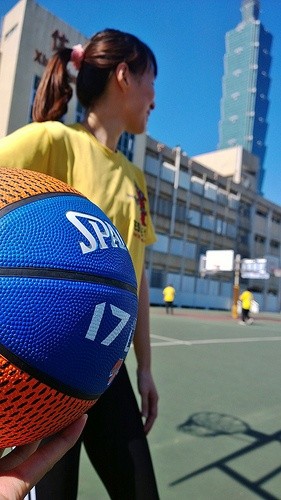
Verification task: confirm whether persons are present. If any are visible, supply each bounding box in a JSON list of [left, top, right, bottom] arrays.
[[163, 282, 175, 316], [0, 26, 161, 500], [235, 287, 254, 326]]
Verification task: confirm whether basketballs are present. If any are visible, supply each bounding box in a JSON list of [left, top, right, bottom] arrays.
[[1, 166, 141, 449]]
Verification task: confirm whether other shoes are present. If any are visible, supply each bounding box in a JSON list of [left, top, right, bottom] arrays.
[[241, 321, 246, 326], [248, 320, 252, 324]]
